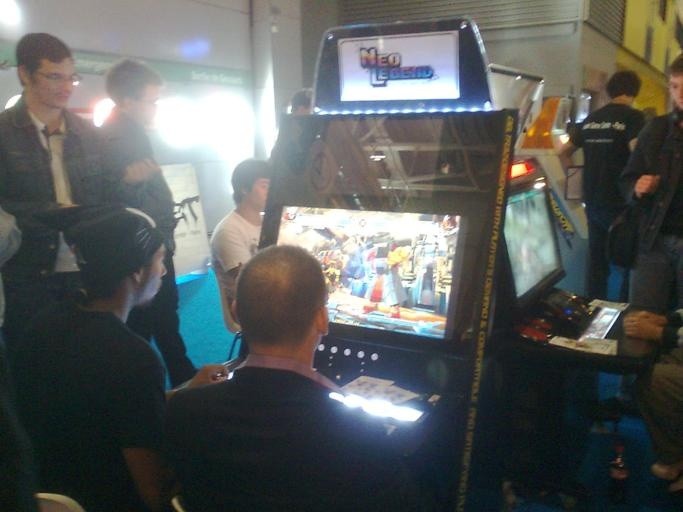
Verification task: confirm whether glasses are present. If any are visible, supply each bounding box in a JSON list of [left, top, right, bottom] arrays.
[[35, 71, 82, 86]]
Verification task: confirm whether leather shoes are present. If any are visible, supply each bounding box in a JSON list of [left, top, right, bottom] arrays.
[[650, 459, 683, 482]]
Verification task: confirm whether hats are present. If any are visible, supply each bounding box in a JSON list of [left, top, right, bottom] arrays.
[[72, 208, 184, 285]]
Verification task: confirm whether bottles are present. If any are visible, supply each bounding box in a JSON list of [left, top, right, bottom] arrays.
[[607, 448, 632, 497]]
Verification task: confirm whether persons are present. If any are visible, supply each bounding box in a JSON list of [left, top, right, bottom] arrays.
[[102, 58, 198, 390], [0, 32, 129, 344], [16, 208, 228, 512], [292, 90, 313, 114], [209, 158, 272, 334], [618, 53, 683, 310], [162, 245, 400, 512], [556, 70, 645, 303], [623, 307, 683, 492]]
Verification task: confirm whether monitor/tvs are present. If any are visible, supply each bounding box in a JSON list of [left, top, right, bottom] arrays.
[[504, 190, 560, 299], [276, 206, 460, 339]]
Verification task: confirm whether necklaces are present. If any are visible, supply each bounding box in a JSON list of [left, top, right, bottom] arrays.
[[613, 98, 633, 103]]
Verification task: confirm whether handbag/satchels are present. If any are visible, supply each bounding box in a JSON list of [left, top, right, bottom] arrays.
[[606, 204, 637, 250]]
